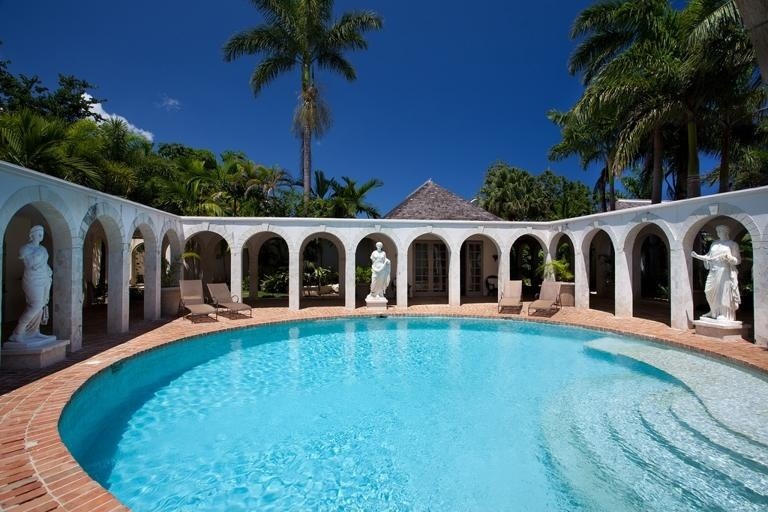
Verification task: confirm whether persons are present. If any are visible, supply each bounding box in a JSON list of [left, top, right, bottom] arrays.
[[9, 224, 54, 342], [369, 241, 391, 296], [691, 224, 742, 320]]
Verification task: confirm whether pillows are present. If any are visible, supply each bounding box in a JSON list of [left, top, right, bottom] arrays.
[[488, 278, 498, 289]]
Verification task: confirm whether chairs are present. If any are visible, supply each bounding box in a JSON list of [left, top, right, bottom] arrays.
[[498, 279, 525, 315], [205, 280, 253, 319], [485, 275, 498, 298], [175, 277, 219, 324], [526, 279, 563, 317]]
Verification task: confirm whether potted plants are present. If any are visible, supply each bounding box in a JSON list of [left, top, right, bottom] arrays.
[[160, 248, 202, 318], [533, 240, 576, 307]]
